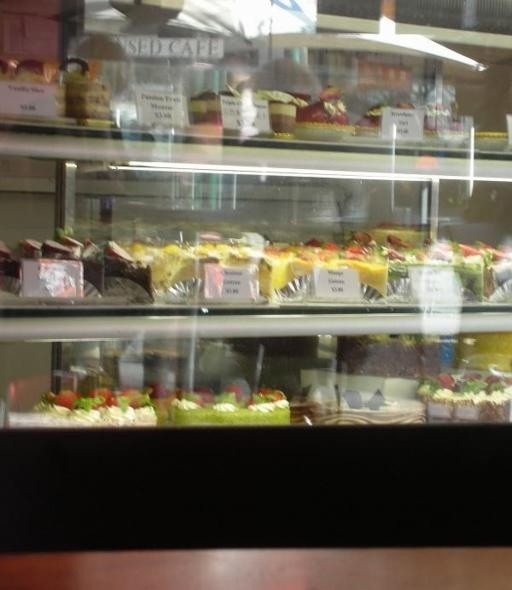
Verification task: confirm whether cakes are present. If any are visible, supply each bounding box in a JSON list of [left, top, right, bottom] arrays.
[[0, 219, 511, 307], [0, 336, 512, 429], [0, 51, 512, 153]]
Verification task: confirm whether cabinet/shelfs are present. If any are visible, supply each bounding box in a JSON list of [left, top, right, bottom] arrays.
[[0, 113, 512, 546]]
